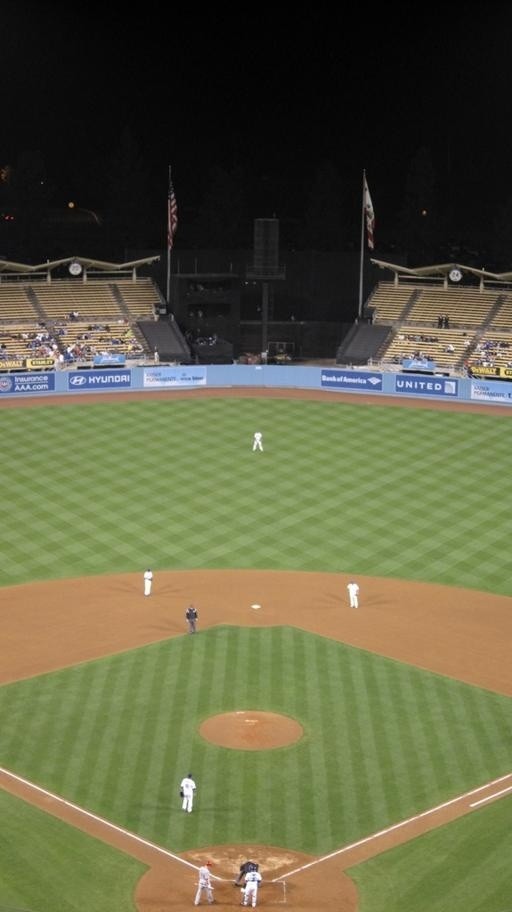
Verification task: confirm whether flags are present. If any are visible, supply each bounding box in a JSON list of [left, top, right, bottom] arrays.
[[167, 176, 179, 251], [364, 177, 376, 250]]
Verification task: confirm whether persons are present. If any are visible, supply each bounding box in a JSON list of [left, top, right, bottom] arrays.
[[180, 773, 197, 813], [194, 860, 215, 906], [243, 873, 261, 906], [346, 581, 361, 610], [0, 311, 160, 365], [144, 569, 153, 599], [251, 428, 267, 452], [185, 606, 198, 635], [391, 314, 512, 378], [235, 862, 259, 885]]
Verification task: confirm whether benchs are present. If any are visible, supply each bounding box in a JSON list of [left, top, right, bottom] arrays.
[[368, 284, 512, 378], [0, 280, 162, 367]]
